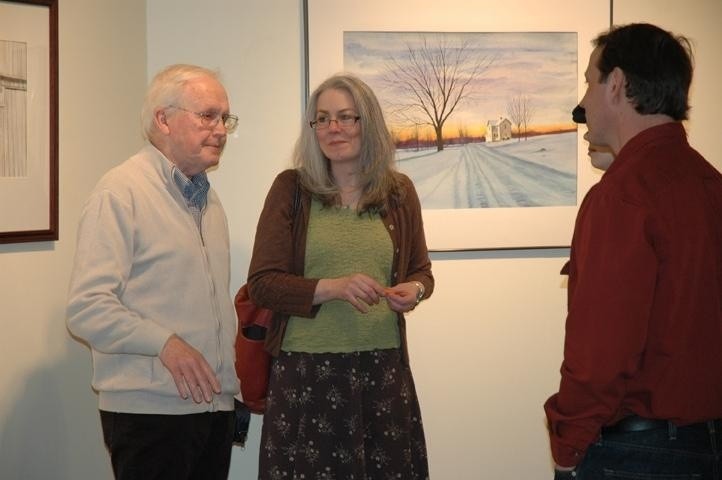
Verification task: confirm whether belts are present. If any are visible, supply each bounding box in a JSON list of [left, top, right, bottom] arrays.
[[600, 409, 717, 433]]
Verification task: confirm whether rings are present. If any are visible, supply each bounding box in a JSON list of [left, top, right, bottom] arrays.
[[409, 305, 413, 312]]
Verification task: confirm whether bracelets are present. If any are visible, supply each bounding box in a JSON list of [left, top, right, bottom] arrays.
[[416, 282, 424, 312]]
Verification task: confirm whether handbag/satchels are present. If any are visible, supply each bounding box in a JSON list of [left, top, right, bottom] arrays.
[[230, 280, 280, 411]]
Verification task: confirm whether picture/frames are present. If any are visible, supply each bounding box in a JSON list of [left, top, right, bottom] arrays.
[[298, 0, 615, 254], [0, 0, 61, 245]]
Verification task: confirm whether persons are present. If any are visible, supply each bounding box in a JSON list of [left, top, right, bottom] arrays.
[[543, 20, 721, 479], [246, 70, 434, 480], [67, 64, 238, 480], [573, 94, 615, 172]]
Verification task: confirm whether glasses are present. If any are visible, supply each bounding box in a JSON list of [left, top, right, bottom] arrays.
[[309, 115, 364, 131], [165, 104, 240, 132]]
